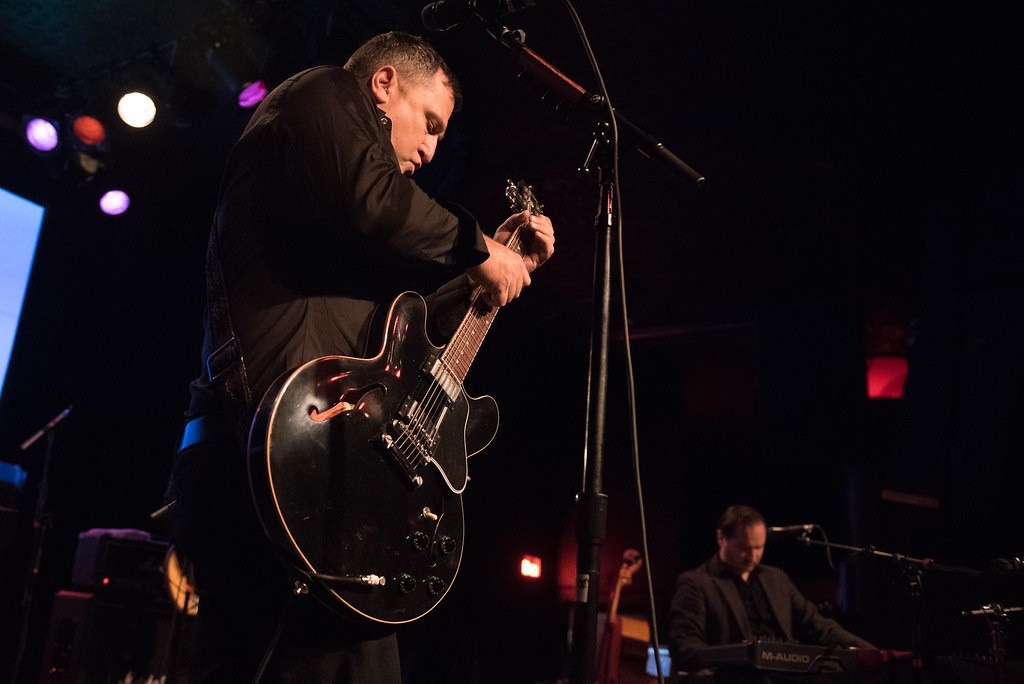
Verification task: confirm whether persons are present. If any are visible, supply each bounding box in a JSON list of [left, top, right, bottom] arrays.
[[615, 548, 653, 620], [166, 27, 561, 683], [656, 503, 882, 684]]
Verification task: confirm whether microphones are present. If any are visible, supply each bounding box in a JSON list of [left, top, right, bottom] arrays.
[[768, 523, 813, 536], [20, 404, 74, 451], [420, 0, 543, 32]]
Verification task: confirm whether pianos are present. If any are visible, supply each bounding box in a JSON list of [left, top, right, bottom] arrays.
[[679, 635, 929, 684]]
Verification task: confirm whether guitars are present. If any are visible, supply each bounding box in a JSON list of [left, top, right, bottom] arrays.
[[244, 175, 545, 635]]
